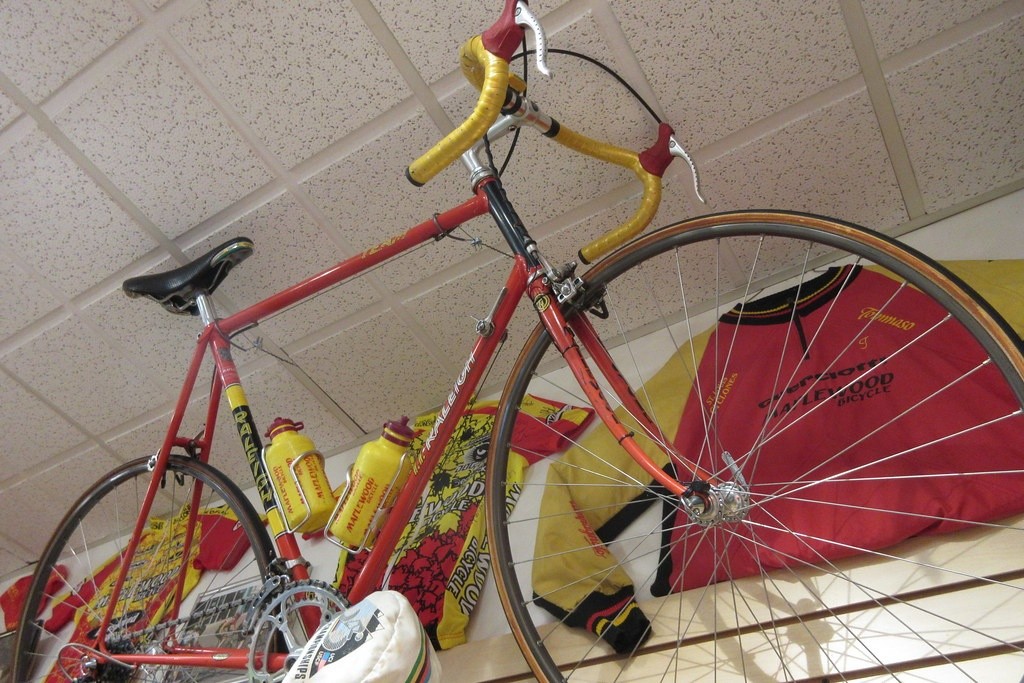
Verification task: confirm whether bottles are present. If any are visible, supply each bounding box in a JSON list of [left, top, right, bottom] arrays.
[[264, 416, 338, 533], [328, 415, 414, 547]]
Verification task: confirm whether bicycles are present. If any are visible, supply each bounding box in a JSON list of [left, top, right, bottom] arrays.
[[7, 0, 1024, 683]]
